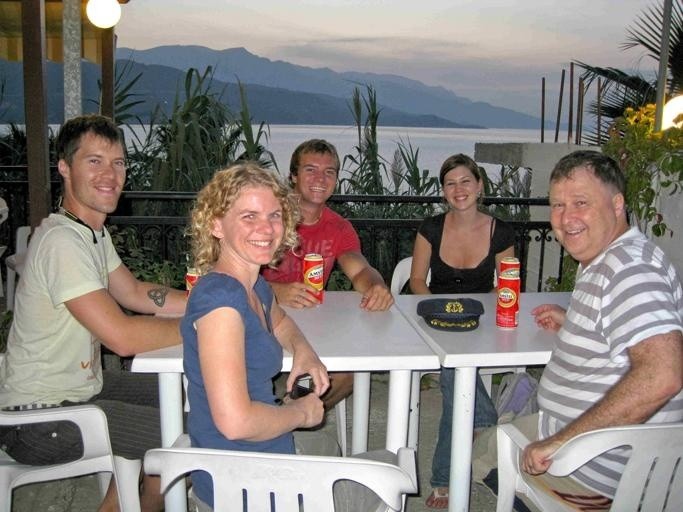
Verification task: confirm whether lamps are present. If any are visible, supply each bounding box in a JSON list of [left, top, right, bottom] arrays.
[[82, 0, 125, 28]]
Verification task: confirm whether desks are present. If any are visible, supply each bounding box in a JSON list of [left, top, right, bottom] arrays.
[[131, 284, 441, 472], [387, 288, 577, 512]]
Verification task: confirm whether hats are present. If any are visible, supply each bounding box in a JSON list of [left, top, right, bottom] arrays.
[[416, 299, 483, 332]]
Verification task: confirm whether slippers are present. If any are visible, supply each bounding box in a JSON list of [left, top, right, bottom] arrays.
[[423, 487, 449, 511]]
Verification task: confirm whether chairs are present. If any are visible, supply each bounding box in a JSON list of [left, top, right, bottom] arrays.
[[387, 252, 529, 403], [140, 437, 421, 512], [0, 197, 11, 298], [295, 371, 349, 456], [5, 225, 31, 314], [0, 350, 144, 512], [492, 421, 682, 512]]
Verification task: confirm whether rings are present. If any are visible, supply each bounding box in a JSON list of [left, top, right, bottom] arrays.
[[295, 294, 301, 301], [363, 295, 369, 300]]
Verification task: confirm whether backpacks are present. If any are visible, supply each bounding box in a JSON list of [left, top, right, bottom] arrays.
[[472, 372, 538, 485]]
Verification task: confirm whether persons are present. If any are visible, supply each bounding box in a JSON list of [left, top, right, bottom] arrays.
[[2, 113, 187, 510], [184, 161, 404, 510], [257, 138, 395, 312], [470, 150, 682, 511], [409, 152, 517, 511]]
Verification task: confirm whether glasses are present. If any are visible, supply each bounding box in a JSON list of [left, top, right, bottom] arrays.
[[53, 196, 104, 243]]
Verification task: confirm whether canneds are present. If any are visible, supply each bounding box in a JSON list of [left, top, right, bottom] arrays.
[[499, 257, 521, 278], [303, 253, 324, 305], [496, 274, 521, 328], [185, 267, 202, 297]]
